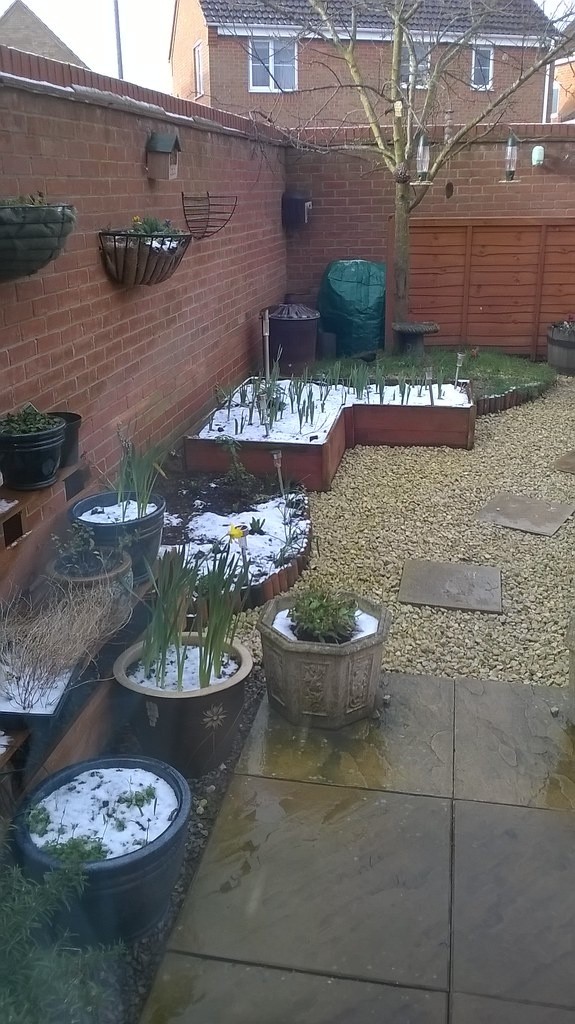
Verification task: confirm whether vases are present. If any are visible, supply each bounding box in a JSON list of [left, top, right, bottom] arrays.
[[98, 232, 194, 286], [48, 412, 82, 467], [546, 328, 575, 377], [111, 629, 255, 778]]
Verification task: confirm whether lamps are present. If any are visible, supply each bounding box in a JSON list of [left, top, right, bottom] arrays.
[[503, 132, 517, 182], [415, 133, 430, 181]]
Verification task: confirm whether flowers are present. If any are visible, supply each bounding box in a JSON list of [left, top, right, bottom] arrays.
[[99, 212, 186, 243], [114, 523, 268, 691], [550, 314, 575, 330]]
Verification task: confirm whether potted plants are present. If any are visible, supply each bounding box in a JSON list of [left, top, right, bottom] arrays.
[[69, 411, 180, 587], [0, 190, 77, 286], [12, 751, 189, 950], [0, 574, 129, 729], [0, 407, 67, 491], [256, 572, 390, 728], [180, 376, 475, 494], [44, 513, 140, 626]]
